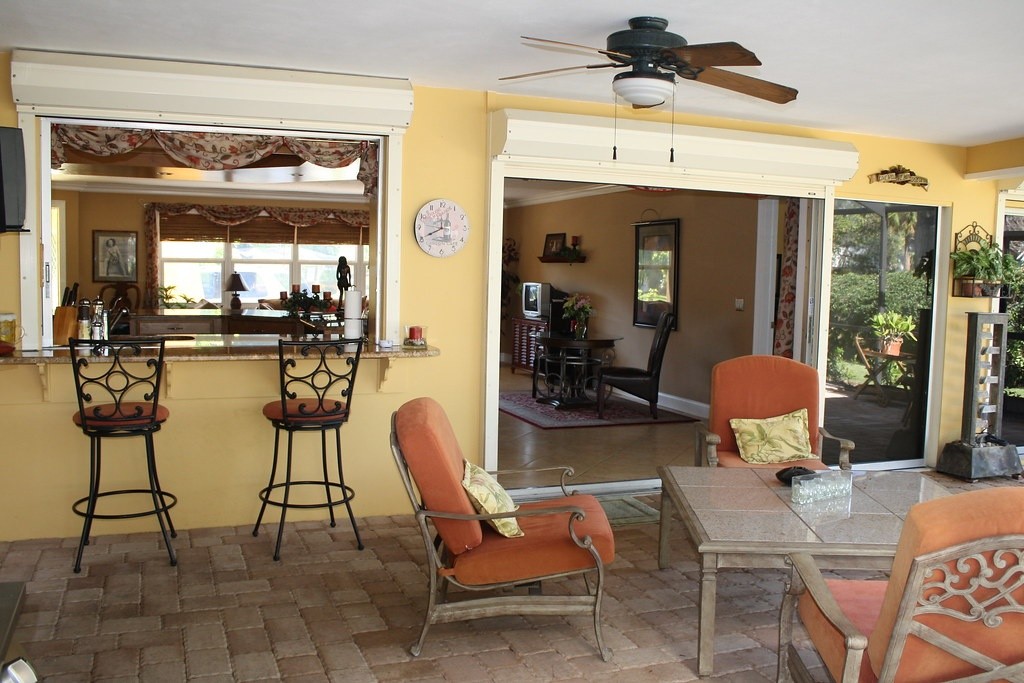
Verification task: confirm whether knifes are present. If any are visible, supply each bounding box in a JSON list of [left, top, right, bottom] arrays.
[[61, 282, 79, 307]]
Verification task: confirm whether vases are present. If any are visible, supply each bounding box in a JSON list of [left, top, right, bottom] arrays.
[[574, 315, 587, 339]]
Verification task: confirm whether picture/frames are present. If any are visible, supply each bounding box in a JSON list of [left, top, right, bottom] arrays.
[[543, 233, 565, 257], [92, 230, 138, 283], [633, 219, 680, 331]]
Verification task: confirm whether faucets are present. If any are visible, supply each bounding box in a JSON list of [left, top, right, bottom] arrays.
[[110, 306, 130, 331]]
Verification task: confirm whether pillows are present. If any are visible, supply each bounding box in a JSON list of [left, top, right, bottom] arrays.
[[462, 457, 524, 538], [729, 408, 819, 464]]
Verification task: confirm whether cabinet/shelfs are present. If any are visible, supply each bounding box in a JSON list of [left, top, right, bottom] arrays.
[[511, 316, 589, 380]]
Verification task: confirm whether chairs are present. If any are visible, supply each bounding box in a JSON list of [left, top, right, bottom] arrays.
[[854, 332, 907, 405], [99, 282, 142, 309], [776, 486, 1024, 683], [695, 355, 855, 468], [597, 311, 675, 419], [252, 339, 364, 561], [69, 336, 178, 573], [390, 397, 616, 662]]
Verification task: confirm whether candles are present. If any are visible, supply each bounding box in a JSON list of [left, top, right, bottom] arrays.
[[572, 236, 578, 245], [323, 292, 331, 300], [280, 291, 287, 300], [292, 284, 300, 293], [312, 285, 320, 293], [409, 327, 422, 339]]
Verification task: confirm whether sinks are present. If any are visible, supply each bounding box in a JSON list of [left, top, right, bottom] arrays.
[[108, 334, 195, 342]]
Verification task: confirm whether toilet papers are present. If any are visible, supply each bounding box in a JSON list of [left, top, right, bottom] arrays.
[[343, 290, 363, 353]]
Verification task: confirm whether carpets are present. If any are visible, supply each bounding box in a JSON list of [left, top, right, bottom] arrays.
[[499, 388, 701, 429]]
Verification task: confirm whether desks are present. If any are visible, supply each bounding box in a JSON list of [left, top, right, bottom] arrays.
[[854, 351, 917, 407], [656, 465, 954, 676], [530, 332, 624, 410]]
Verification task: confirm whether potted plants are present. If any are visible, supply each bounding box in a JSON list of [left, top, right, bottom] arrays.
[[950, 243, 1024, 297], [870, 310, 917, 355]]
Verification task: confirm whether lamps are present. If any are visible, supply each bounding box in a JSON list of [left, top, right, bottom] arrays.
[[222, 271, 248, 309], [611, 56, 676, 105]]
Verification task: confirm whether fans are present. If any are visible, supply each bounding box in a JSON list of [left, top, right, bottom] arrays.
[[497, 16, 798, 104]]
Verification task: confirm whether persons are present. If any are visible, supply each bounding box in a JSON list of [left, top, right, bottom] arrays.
[[104, 238, 127, 276], [336, 256, 352, 306]]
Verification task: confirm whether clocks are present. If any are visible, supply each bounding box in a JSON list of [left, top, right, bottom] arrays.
[[415, 198, 470, 257]]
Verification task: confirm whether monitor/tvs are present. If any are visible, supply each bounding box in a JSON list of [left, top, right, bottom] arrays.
[[522, 282, 569, 321], [0, 126, 26, 226]]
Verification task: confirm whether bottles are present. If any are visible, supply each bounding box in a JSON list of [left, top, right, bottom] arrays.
[[78, 294, 108, 346]]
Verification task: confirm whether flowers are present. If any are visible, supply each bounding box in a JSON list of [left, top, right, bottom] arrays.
[[562, 292, 592, 319]]
